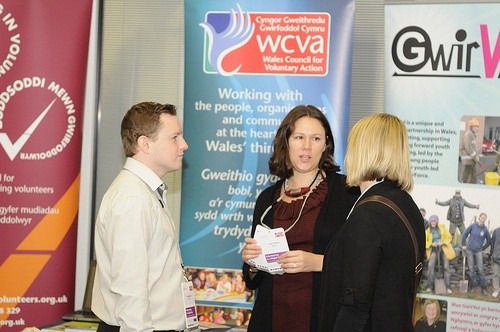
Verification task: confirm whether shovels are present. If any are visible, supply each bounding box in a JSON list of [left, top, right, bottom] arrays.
[[459, 253, 468, 292], [435, 247, 446, 295]]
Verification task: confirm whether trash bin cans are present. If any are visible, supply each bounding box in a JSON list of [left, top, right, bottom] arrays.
[[483, 172, 499, 186]]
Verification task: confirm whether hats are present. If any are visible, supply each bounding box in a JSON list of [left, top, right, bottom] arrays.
[[455, 189, 460, 194], [429, 215, 438, 224]]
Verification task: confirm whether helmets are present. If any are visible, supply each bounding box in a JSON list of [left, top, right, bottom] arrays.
[[469, 118, 479, 127]]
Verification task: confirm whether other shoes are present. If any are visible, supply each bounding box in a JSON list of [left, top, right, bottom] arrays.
[[426, 288, 431, 293], [447, 288, 452, 294], [469, 286, 475, 292], [491, 292, 498, 297], [482, 288, 488, 295]]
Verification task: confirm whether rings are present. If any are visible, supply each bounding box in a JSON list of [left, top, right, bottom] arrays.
[[294, 263, 296, 268]]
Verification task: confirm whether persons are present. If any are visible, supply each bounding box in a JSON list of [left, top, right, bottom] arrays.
[[322, 113, 425, 332], [91, 101, 199, 332], [186, 268, 256, 303], [419, 191, 500, 296], [241, 105, 362, 332], [461, 119, 480, 183], [415, 298, 447, 332]]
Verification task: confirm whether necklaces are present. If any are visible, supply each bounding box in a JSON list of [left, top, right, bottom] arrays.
[[284, 170, 320, 197]]
